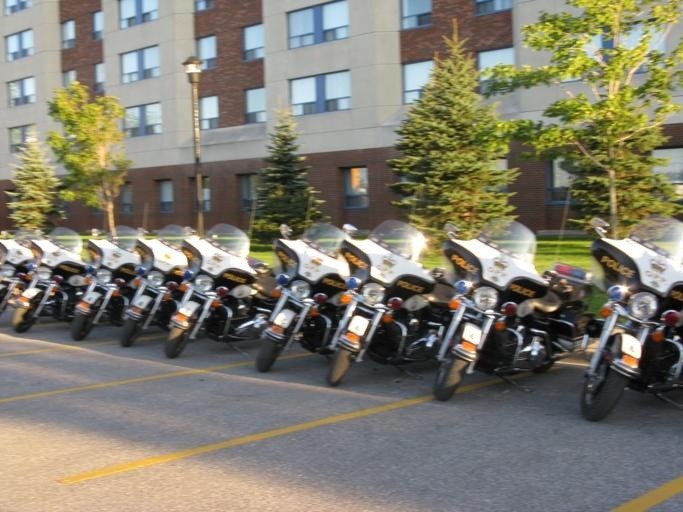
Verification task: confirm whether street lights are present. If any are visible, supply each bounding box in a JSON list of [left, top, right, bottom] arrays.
[[179, 56, 207, 238]]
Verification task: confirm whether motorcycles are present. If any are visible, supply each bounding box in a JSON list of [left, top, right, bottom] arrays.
[[163, 220, 279, 360], [432, 215, 594, 402], [578, 213, 682, 423], [253, 220, 362, 372], [326, 218, 457, 386], [0, 223, 191, 350]]
